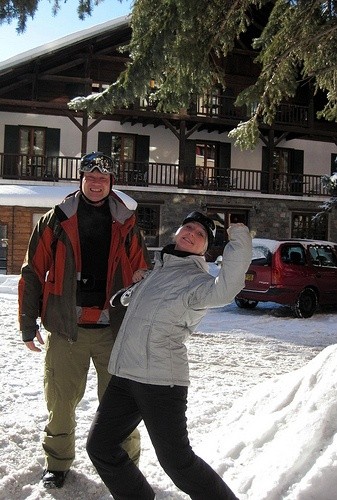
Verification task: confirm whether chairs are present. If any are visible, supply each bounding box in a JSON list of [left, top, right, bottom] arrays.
[[289, 252, 301, 264]]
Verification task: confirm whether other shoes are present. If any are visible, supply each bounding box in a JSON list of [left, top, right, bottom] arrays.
[[45, 470, 65, 487]]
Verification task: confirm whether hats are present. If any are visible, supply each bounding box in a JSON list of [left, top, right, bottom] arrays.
[[79, 152, 114, 173], [181, 211, 218, 236]]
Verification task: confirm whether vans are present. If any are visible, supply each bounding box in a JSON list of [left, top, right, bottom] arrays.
[[235, 239, 337, 318]]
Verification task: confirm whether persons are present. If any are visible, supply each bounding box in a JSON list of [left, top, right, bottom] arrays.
[[18, 151, 152, 490], [85, 211, 253, 500]]
